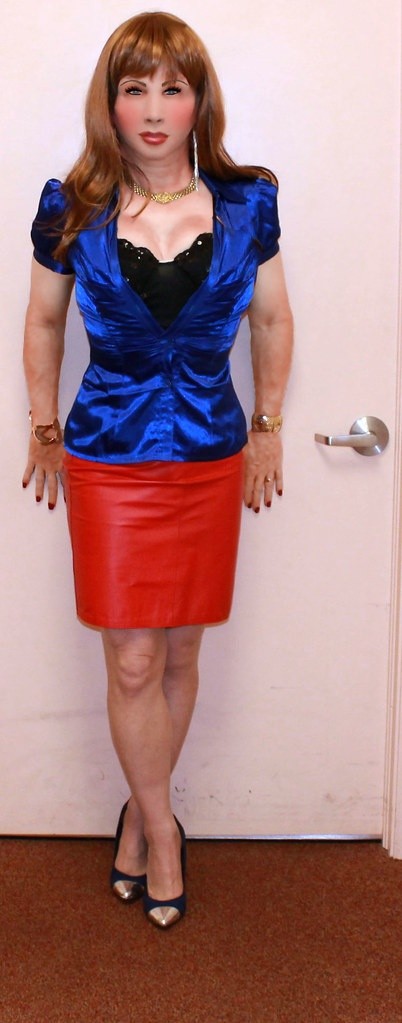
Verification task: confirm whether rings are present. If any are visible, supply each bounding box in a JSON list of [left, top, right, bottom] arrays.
[[265, 477, 274, 482]]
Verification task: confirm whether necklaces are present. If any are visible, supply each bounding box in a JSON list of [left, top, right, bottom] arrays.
[[127, 177, 196, 204]]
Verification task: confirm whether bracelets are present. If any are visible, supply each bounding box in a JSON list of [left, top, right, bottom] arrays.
[[28, 410, 62, 445], [252, 414, 282, 432]]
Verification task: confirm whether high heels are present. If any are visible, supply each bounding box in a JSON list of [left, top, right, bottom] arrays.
[[110, 799, 148, 900], [143, 813, 187, 928]]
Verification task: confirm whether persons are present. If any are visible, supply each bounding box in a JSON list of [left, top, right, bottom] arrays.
[[23, 12, 294, 927]]
